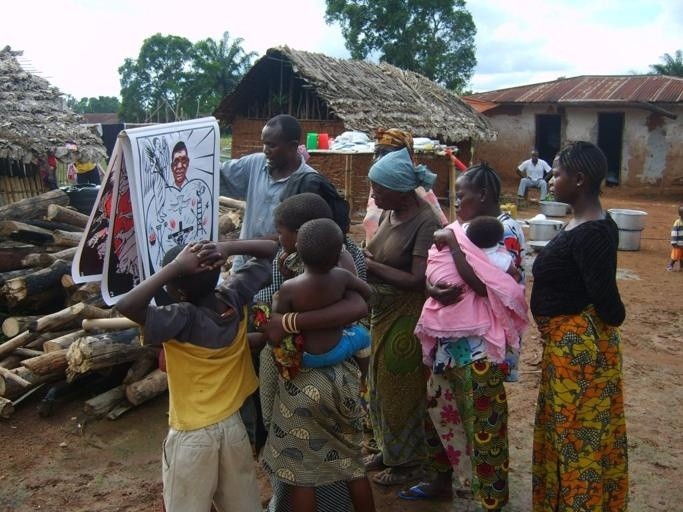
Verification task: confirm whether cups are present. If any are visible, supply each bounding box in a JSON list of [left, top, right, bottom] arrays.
[[306, 133, 317, 149], [318, 134, 328, 149], [430, 140, 447, 155]]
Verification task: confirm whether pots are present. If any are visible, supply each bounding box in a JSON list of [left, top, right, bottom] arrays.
[[526, 219, 562, 241], [618, 230, 641, 250], [538, 200, 568, 217], [605, 208, 648, 231]]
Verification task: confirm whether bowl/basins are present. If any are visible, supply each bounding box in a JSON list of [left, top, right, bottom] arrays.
[[525, 240, 549, 251]]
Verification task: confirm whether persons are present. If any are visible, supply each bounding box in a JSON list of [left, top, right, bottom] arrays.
[[262, 191, 382, 511], [358, 148, 455, 501], [420, 160, 532, 511], [462, 217, 521, 284], [245, 169, 365, 347], [260, 217, 371, 387], [169, 142, 189, 191], [513, 149, 553, 202], [529, 139, 631, 511], [361, 125, 448, 474], [663, 206, 682, 273], [212, 113, 345, 355], [32, 137, 103, 195], [112, 238, 279, 511]]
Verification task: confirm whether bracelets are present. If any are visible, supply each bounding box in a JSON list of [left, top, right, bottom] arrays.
[[281, 312, 291, 334], [293, 312, 301, 334], [287, 312, 296, 333]]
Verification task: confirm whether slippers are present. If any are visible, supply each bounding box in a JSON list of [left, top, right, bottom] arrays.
[[363, 453, 386, 471], [372, 467, 426, 487], [396, 480, 454, 501]]
